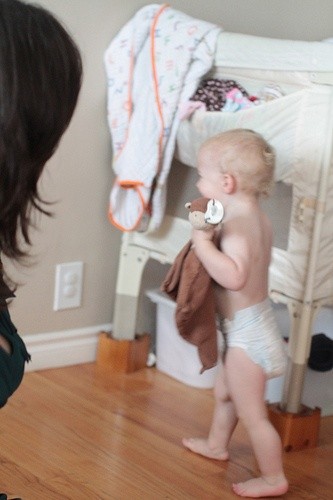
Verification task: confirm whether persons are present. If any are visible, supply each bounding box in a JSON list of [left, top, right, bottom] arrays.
[[192, 129, 291, 497], [0, 0, 83, 500]]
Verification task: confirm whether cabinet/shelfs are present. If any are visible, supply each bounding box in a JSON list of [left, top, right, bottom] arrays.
[[94, 3, 333, 450]]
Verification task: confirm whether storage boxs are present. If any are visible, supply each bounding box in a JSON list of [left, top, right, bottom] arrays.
[[145, 281, 229, 391]]
